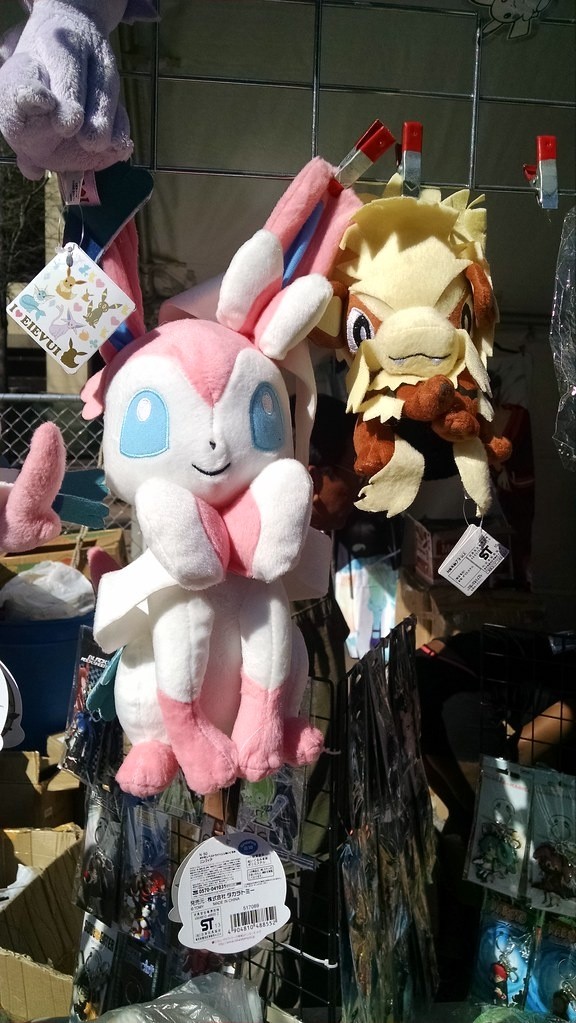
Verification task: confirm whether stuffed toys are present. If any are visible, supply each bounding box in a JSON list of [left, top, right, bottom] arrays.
[[306, 190, 494, 517], [0, 0, 134, 180], [0, 422, 67, 553], [82, 230, 334, 797]]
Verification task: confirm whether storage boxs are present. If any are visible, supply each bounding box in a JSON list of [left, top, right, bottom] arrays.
[[0, 526, 125, 586], [0, 821, 85, 1023], [46, 732, 65, 764], [0, 749, 83, 829]]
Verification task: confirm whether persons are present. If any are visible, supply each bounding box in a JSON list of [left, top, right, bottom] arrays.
[[478, 369, 537, 592], [196, 392, 367, 1006], [414, 629, 576, 883]]
